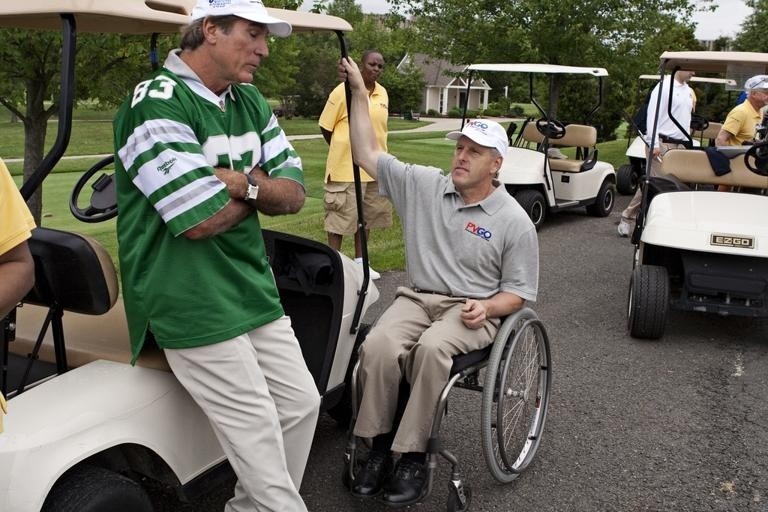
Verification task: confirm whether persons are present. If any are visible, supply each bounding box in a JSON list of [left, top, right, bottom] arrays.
[[0, 160, 38, 433], [338, 56, 541, 507], [112, 0, 321, 512], [318, 51, 393, 280], [714, 74, 768, 192], [617, 69, 697, 238]]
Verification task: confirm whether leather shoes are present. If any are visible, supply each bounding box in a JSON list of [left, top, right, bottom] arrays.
[[381, 453, 428, 508], [350, 452, 394, 497]]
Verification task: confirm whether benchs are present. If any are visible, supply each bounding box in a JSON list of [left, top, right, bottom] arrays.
[[520, 122, 599, 174], [675, 121, 724, 146], [2, 225, 175, 371]]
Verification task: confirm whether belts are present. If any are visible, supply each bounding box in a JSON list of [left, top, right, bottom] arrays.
[[659, 134, 675, 142]]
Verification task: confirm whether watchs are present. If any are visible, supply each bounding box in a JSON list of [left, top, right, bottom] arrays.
[[244, 174, 259, 202]]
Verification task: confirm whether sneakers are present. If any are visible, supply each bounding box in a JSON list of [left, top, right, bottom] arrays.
[[353, 257, 381, 280], [617, 218, 630, 237]]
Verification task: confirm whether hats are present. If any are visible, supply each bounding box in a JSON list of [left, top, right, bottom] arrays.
[[445, 118, 508, 159], [189, 0, 292, 38], [744, 75, 768, 89]]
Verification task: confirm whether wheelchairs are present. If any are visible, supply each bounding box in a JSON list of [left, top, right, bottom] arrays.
[[343, 294, 560, 509]]
